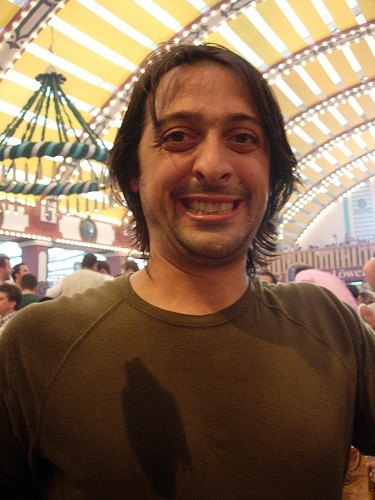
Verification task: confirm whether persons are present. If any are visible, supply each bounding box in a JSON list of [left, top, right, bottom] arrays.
[[1, 44, 375, 500], [254, 257, 375, 322], [0, 252, 140, 332]]
